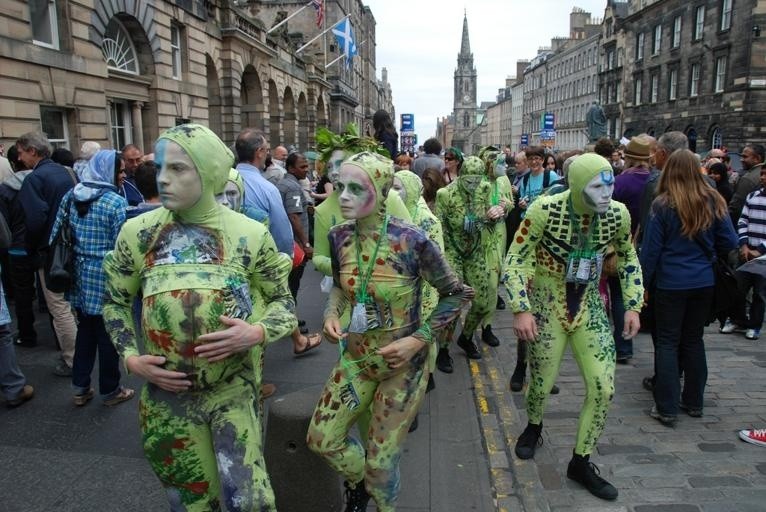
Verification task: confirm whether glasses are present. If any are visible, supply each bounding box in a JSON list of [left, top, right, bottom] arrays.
[[445, 156, 454, 161]]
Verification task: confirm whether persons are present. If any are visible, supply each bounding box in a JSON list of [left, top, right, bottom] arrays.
[[591, 131, 765, 444], [386, 135, 582, 395], [501, 153, 645, 501], [102, 125, 300, 512], [586, 99, 609, 143], [0, 129, 163, 405], [216, 126, 423, 355], [304, 149, 466, 512]]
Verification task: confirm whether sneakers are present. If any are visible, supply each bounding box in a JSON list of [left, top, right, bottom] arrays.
[[53, 360, 74, 377], [298, 319, 306, 327], [262, 383, 276, 398], [719, 317, 739, 334], [566, 447, 619, 499], [103, 385, 136, 407], [497, 294, 506, 310], [438, 348, 454, 373], [299, 327, 308, 334], [457, 331, 482, 359], [514, 421, 544, 459], [481, 324, 499, 347], [12, 337, 38, 348], [509, 361, 527, 391], [643, 377, 702, 424], [342, 478, 372, 512], [7, 385, 34, 406], [739, 429, 766, 447], [73, 388, 95, 406], [746, 326, 760, 340]]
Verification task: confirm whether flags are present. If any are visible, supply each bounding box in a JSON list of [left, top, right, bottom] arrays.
[[312, 1, 325, 30], [330, 16, 359, 64]]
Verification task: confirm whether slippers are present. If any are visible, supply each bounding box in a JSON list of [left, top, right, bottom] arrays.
[[294, 334, 322, 354]]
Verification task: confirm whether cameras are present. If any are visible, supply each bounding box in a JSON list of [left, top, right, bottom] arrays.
[[522, 195, 530, 203]]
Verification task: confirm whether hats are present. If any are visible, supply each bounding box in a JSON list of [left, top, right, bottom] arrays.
[[622, 136, 655, 160]]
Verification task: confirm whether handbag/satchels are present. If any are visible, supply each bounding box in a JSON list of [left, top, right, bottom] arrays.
[[715, 263, 738, 313], [44, 187, 75, 293]]
